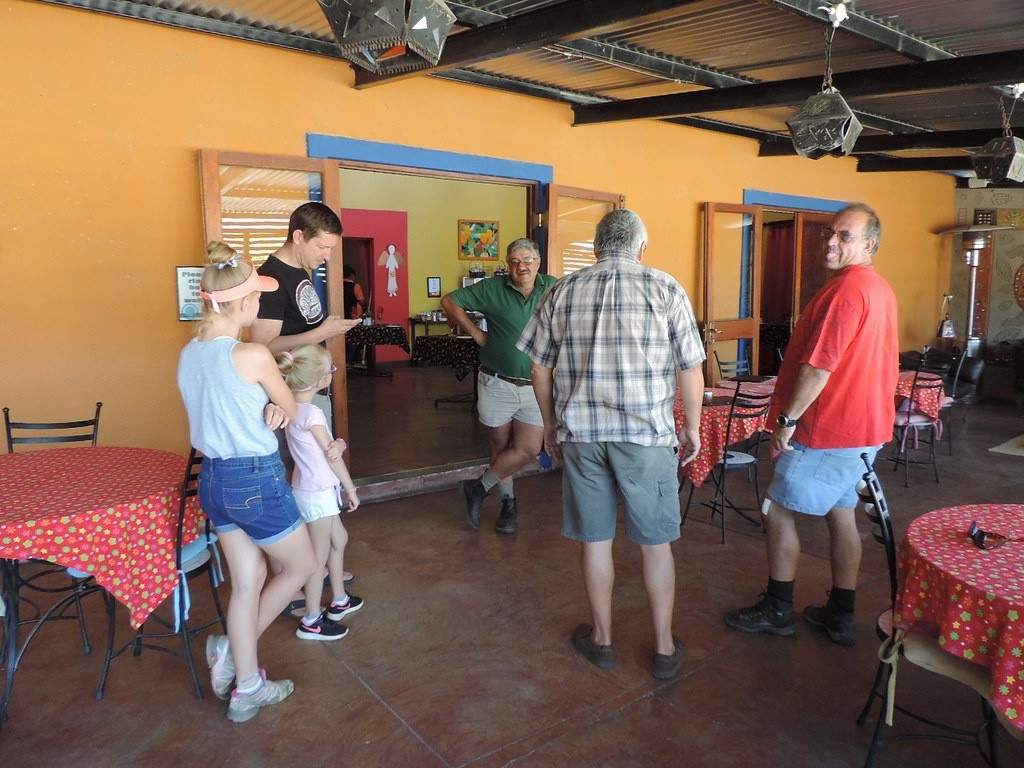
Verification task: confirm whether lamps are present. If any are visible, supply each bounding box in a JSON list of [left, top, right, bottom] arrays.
[[786, 4, 863, 160], [315, 0, 455, 76], [936, 224, 1016, 358], [971, 83, 1024, 183]]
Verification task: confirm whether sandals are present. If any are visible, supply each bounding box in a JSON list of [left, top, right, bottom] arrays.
[[323, 569, 355, 586], [281, 599, 328, 620]]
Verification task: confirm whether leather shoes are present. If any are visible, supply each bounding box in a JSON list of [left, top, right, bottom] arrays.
[[458, 479, 490, 529], [496, 494, 517, 533]]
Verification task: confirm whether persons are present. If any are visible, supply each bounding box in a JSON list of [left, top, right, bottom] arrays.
[[177, 241, 319, 723], [273, 343, 363, 640], [724, 202, 900, 645], [343, 263, 366, 371], [442, 237, 558, 531], [515, 209, 707, 679], [249, 202, 363, 621]]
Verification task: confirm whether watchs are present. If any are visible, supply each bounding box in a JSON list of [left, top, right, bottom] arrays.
[[775, 412, 797, 427]]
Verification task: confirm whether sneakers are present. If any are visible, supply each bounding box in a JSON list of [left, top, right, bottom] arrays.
[[206, 633, 236, 700], [725, 589, 794, 636], [327, 590, 363, 621], [227, 668, 294, 723], [803, 589, 857, 646], [295, 613, 349, 641]]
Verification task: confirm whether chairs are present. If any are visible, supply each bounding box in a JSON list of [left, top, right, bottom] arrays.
[[888, 346, 983, 488], [680, 383, 777, 542], [713, 350, 751, 377], [853, 452, 1000, 768], [0, 400, 107, 666], [95, 447, 227, 701]]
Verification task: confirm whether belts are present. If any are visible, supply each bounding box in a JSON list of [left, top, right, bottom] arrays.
[[479, 366, 532, 386]]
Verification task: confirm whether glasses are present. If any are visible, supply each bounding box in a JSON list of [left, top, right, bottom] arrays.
[[819, 229, 869, 243], [316, 365, 337, 386], [507, 256, 538, 266], [969, 521, 1024, 550]]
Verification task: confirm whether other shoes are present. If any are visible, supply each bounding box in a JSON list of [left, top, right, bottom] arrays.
[[575, 624, 615, 669], [652, 634, 685, 678]]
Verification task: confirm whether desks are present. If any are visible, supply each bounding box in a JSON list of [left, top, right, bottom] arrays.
[[0, 446, 211, 721], [408, 317, 459, 363], [901, 503, 1024, 732], [345, 323, 411, 379], [894, 370, 944, 470], [414, 332, 479, 414], [672, 375, 778, 529]]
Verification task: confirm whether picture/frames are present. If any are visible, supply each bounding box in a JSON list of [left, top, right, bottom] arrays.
[[457, 219, 501, 261], [176, 265, 210, 322], [427, 277, 441, 297]]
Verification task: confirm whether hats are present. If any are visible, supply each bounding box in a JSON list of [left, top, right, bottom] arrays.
[[200, 260, 279, 314]]
